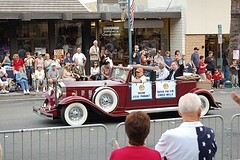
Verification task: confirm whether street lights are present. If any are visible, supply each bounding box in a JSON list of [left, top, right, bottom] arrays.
[[119, 0, 133, 65]]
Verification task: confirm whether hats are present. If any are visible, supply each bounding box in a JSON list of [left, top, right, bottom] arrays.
[[194, 47, 199, 50], [136, 68, 143, 73], [51, 63, 56, 67]]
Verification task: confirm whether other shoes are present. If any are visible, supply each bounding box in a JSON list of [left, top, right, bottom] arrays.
[[27, 90, 30, 94], [24, 91, 27, 95], [232, 86, 236, 88], [0, 90, 10, 94], [43, 89, 46, 93], [236, 86, 239, 89], [36, 90, 39, 93]]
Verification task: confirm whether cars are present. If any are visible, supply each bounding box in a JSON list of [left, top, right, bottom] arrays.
[[32, 63, 223, 127]]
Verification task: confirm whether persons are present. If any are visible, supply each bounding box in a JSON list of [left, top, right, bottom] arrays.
[[99, 33, 160, 59], [24, 52, 35, 72], [165, 61, 182, 80], [12, 53, 26, 75], [156, 61, 170, 81], [154, 93, 217, 160], [23, 35, 73, 53], [43, 54, 54, 72], [90, 61, 101, 80], [63, 52, 73, 67], [89, 40, 100, 74], [51, 55, 61, 68], [34, 51, 44, 70], [16, 66, 30, 95], [0, 63, 9, 94], [48, 64, 59, 90], [190, 47, 240, 90], [35, 64, 46, 93], [133, 45, 189, 72], [109, 111, 162, 160], [72, 59, 88, 81], [72, 47, 87, 77], [101, 49, 114, 68], [2, 51, 11, 67], [62, 64, 74, 81], [132, 68, 146, 83], [100, 62, 112, 80]]
